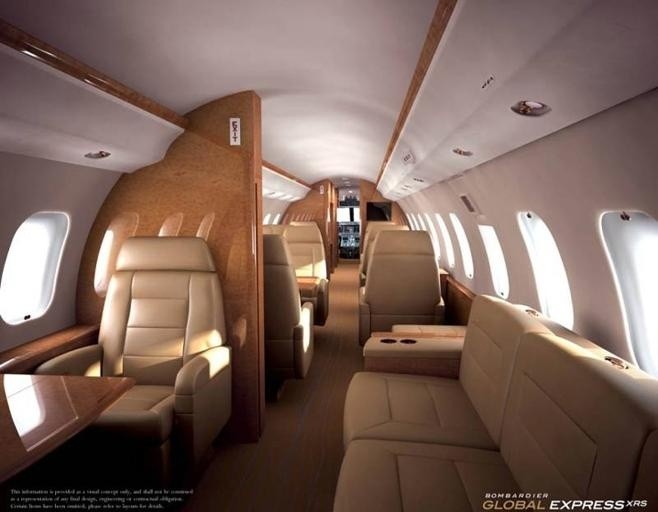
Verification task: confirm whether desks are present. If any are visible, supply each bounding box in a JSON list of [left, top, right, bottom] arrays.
[[0, 368, 138, 495]]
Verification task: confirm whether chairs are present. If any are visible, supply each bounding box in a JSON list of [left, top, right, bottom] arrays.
[[334, 219, 656, 511], [34, 232, 234, 478], [260, 218, 330, 404]]
